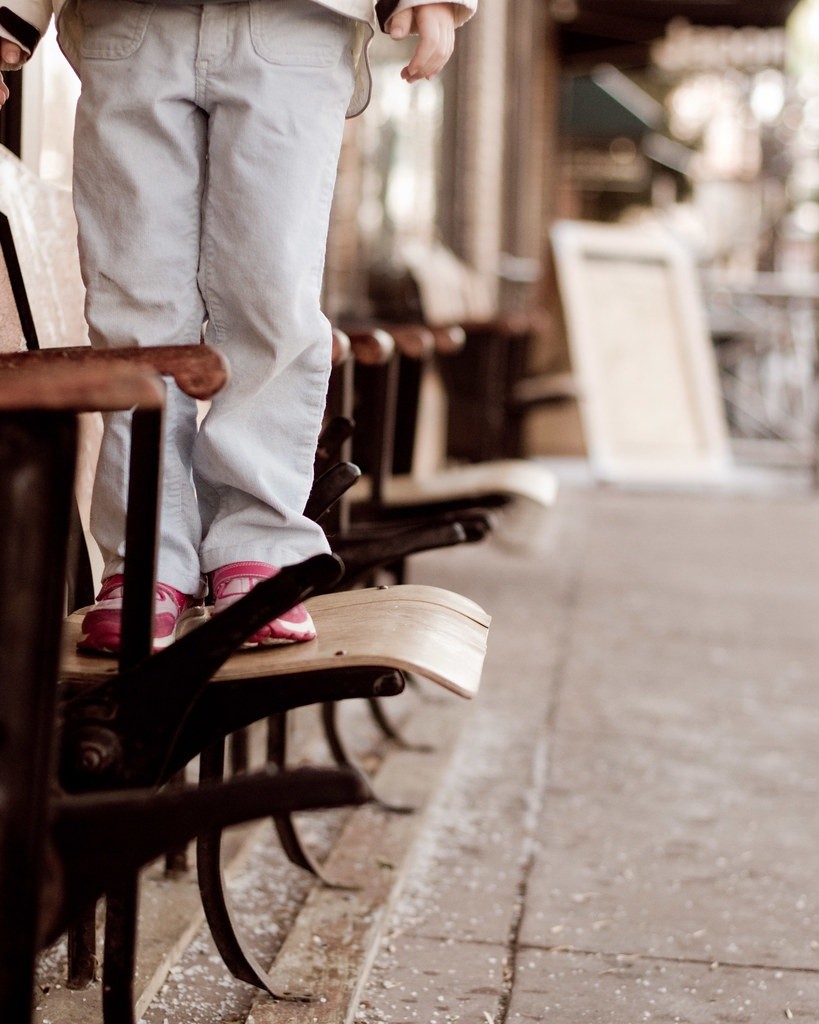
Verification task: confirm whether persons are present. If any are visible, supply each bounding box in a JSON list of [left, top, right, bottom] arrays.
[[0, 0, 482, 655]]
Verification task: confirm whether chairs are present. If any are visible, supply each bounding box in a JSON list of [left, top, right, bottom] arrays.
[[1, 141, 587, 1024]]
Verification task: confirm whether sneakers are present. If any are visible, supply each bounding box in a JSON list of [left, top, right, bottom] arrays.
[[77, 573, 205, 657], [216, 563, 317, 648]]
